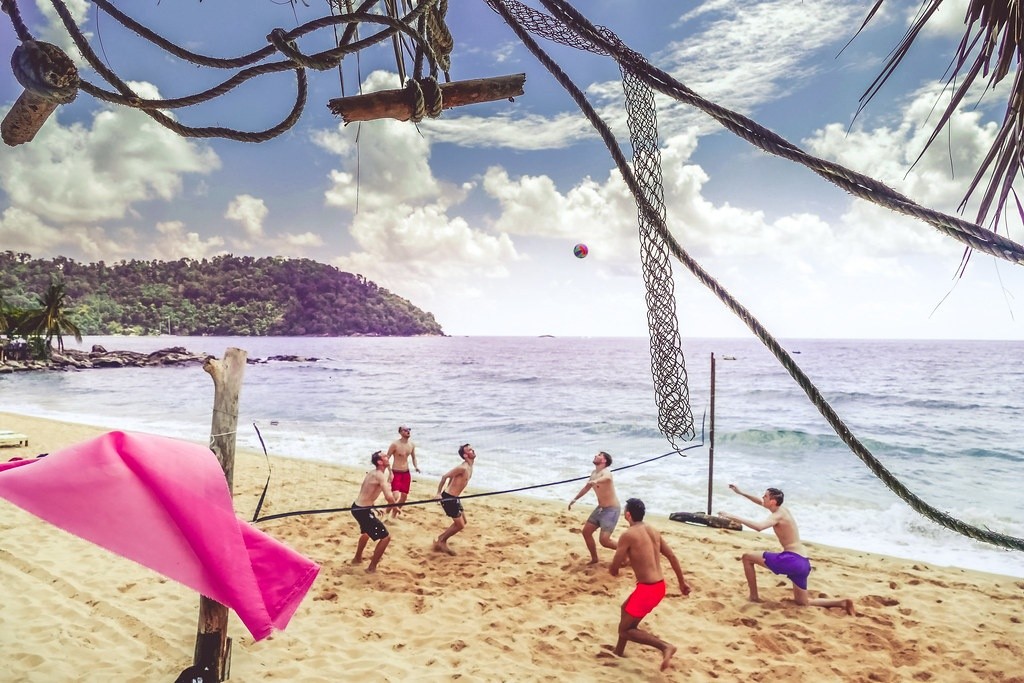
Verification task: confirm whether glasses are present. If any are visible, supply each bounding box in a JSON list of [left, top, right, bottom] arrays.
[[400, 428, 411, 432]]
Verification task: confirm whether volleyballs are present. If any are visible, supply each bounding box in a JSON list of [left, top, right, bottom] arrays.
[[573, 243, 589, 259]]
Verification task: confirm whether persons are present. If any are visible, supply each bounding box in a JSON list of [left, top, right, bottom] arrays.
[[610, 498, 691, 671], [350, 451, 400, 574], [386, 426, 421, 518], [568, 452, 621, 565], [434, 444, 476, 556], [718, 483, 856, 617]]
